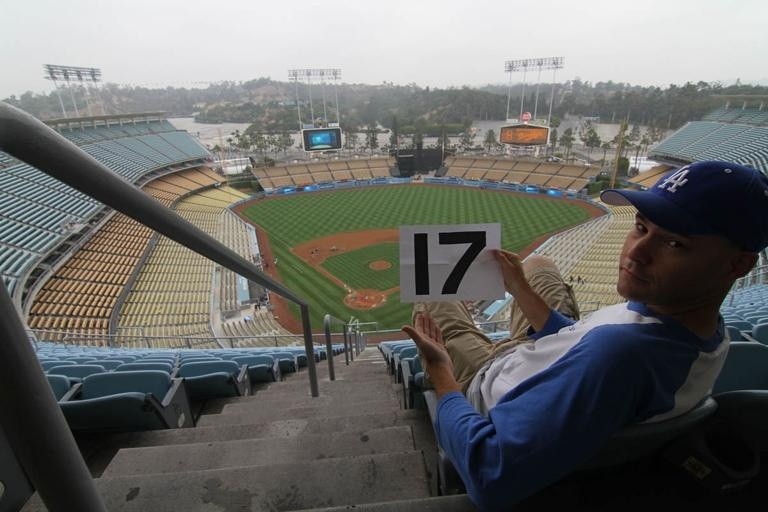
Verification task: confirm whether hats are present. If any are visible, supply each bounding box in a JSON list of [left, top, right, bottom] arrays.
[[597, 159, 768, 253]]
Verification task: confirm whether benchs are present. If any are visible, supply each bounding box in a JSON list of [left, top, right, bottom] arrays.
[[1, 313, 768, 512]]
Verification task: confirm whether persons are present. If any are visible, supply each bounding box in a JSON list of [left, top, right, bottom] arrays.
[[403, 160, 768, 512]]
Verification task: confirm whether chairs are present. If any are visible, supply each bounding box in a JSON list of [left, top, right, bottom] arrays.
[[1, 115, 297, 336], [485, 163, 766, 312], [647, 107, 768, 163], [298, 150, 402, 191], [423, 152, 602, 197]]
